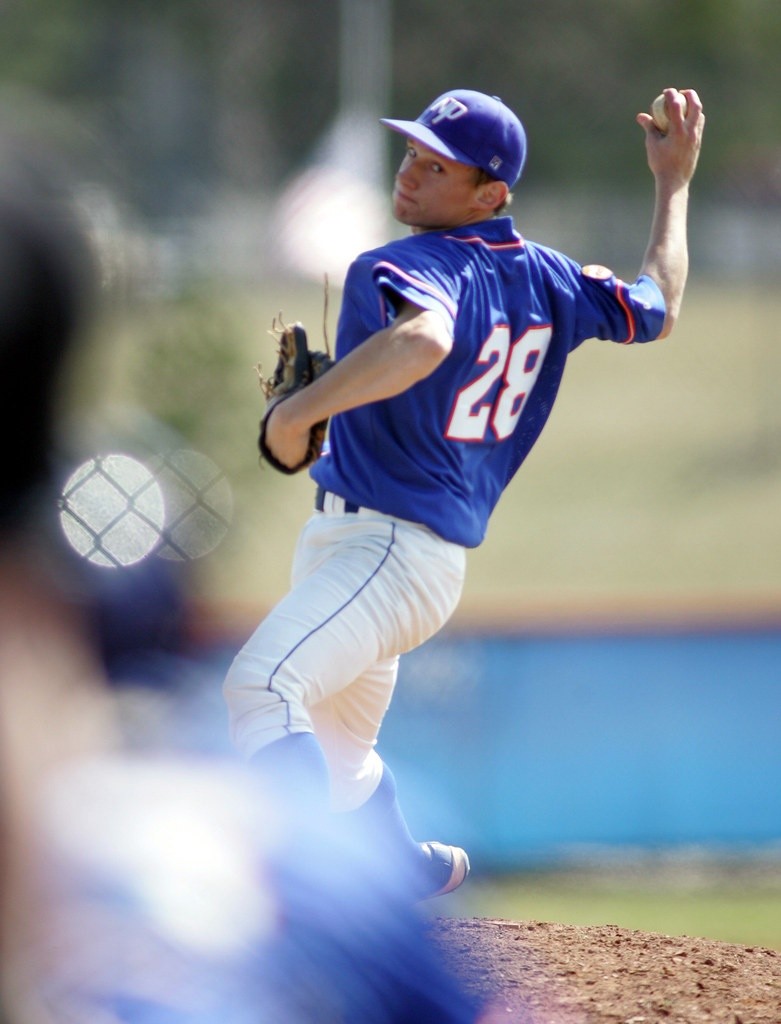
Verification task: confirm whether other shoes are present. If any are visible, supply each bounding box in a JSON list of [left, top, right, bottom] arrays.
[[402, 840, 471, 904]]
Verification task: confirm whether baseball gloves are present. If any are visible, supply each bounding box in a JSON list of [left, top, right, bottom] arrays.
[[252, 312, 338, 475]]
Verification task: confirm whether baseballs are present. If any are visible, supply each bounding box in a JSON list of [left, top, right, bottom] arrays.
[[651, 88, 687, 135]]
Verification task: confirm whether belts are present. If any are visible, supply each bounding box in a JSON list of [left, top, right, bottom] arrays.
[[314, 485, 358, 514]]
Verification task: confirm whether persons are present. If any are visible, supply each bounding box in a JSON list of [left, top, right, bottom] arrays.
[[0, 151, 489, 1024], [224, 87, 704, 895]]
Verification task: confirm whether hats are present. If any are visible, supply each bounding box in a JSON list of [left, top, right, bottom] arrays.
[[379, 88, 527, 188]]
